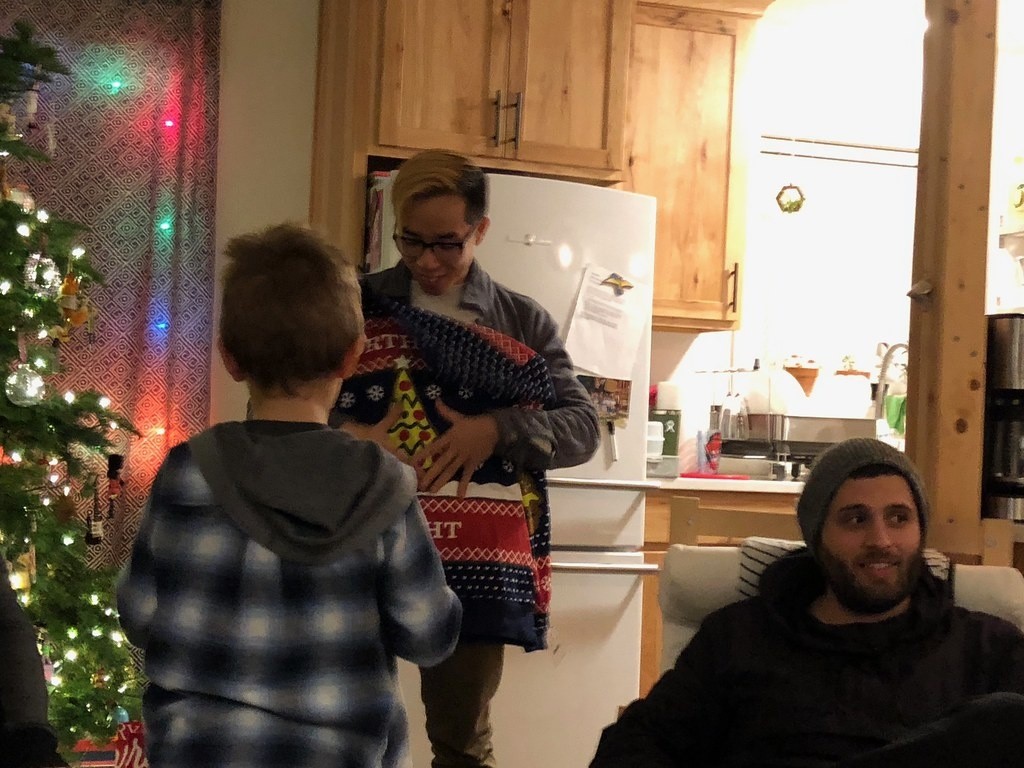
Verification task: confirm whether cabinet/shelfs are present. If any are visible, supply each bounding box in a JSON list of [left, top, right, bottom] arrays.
[[308, 0, 776, 334], [643, 489, 802, 696]]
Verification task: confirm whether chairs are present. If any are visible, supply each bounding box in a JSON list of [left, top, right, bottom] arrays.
[[662, 496, 1024, 672]]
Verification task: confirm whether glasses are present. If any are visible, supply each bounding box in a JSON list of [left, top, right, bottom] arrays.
[[392, 221, 481, 257]]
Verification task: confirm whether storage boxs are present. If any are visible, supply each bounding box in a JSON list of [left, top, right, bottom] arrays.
[[647, 455, 679, 478]]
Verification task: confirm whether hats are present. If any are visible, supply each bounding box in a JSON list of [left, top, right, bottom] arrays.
[[799, 437, 925, 560]]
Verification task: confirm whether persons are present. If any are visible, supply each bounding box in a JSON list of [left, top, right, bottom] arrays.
[[246, 148, 600, 768], [0, 556, 69, 768], [117, 221, 464, 767], [589, 438, 1024, 768]]
